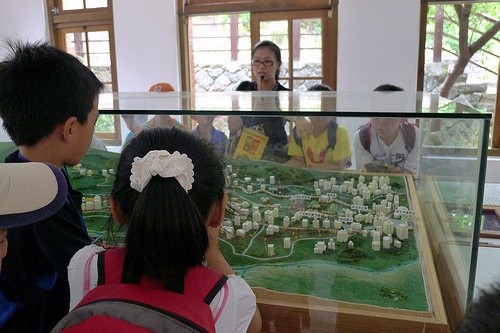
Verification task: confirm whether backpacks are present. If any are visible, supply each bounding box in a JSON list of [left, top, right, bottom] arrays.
[[51, 247, 229, 333]]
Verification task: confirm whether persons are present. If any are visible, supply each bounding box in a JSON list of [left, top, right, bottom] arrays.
[[0, 38, 105, 333], [227, 40, 292, 163], [354, 84, 420, 179], [189, 115, 229, 156], [121, 115, 148, 149], [144, 83, 188, 131], [67, 127, 263, 333], [282, 84, 352, 171], [0, 160, 68, 333]]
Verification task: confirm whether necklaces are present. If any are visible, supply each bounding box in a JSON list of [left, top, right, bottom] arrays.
[[376, 125, 409, 168]]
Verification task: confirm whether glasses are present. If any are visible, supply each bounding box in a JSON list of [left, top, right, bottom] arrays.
[[251, 59, 278, 67]]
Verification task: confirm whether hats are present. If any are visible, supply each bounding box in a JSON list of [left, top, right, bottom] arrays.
[[2, 162, 68, 229], [149, 83, 175, 92]]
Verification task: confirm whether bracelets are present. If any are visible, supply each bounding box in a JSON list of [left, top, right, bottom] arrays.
[[294, 116, 298, 122]]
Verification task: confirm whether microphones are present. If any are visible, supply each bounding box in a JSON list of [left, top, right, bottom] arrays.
[[260, 75, 264, 91]]
